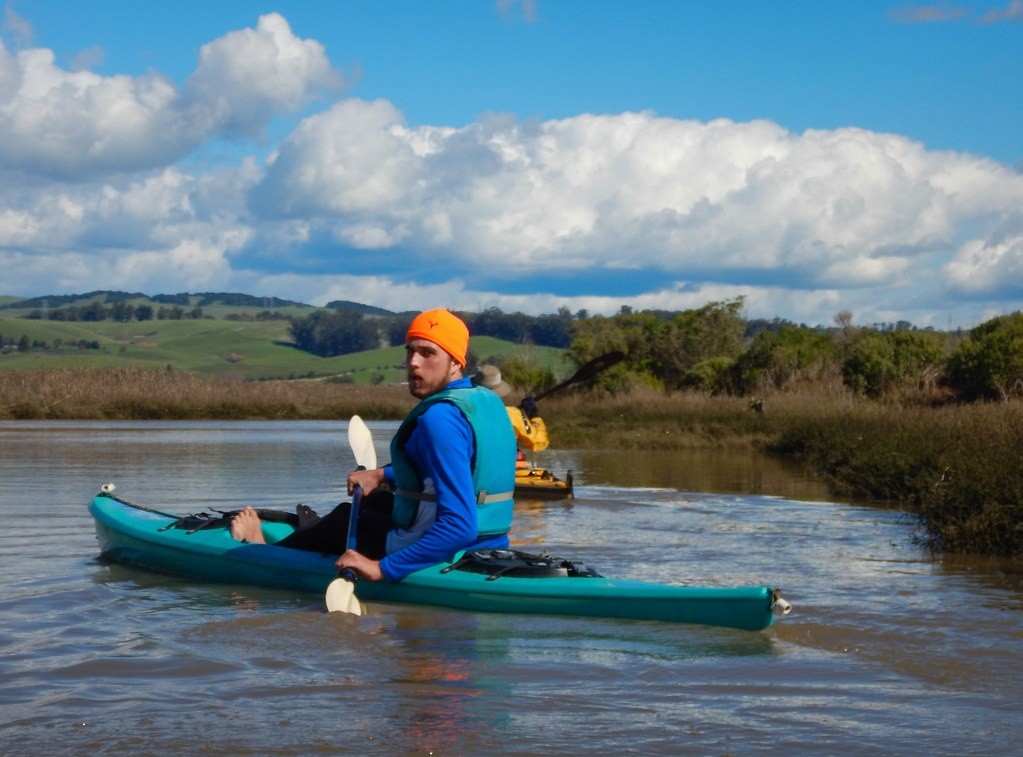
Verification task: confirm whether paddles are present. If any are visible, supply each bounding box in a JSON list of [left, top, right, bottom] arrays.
[[324, 414, 380, 615], [512, 347, 628, 409]]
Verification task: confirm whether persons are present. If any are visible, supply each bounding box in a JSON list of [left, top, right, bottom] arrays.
[[230, 308, 516, 581], [468, 366, 549, 452]]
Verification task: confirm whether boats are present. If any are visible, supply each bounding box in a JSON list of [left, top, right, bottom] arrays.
[[88, 476, 791, 635], [508, 450, 576, 501]]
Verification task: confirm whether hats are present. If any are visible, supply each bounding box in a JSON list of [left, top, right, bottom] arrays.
[[405, 307, 469, 368], [466, 365, 512, 398]]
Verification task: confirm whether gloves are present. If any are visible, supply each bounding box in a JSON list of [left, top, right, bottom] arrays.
[[521, 396, 538, 421]]
